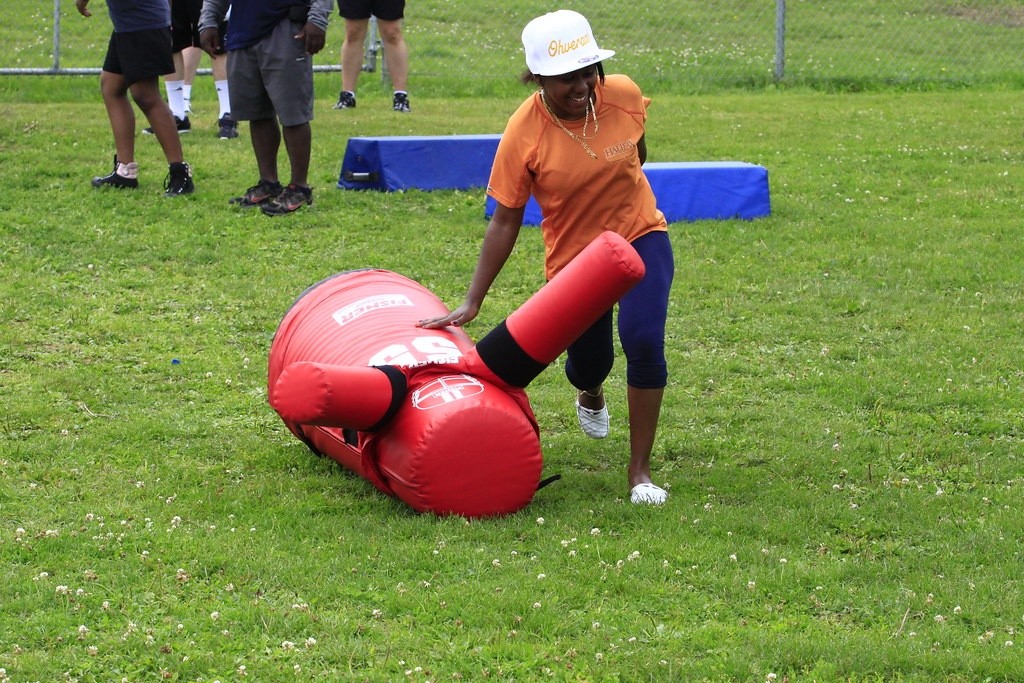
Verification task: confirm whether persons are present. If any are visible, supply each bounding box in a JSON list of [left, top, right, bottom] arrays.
[[413, 10, 675, 506], [197, 0, 334, 215], [168, 0, 240, 140], [331, 0, 412, 113], [142, 1, 192, 137], [75, 0, 194, 198]]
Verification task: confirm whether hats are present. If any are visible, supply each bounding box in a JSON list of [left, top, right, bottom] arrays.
[[520, 10, 616, 75]]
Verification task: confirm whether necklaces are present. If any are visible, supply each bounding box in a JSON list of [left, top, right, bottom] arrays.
[[541, 87, 599, 160]]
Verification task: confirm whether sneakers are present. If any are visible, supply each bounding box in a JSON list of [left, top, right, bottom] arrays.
[[91, 154, 139, 189], [230, 181, 285, 209], [261, 185, 314, 217], [162, 161, 194, 198], [218, 113, 239, 141], [142, 115, 190, 135]]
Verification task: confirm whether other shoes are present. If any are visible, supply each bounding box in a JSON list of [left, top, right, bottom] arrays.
[[331, 91, 355, 110], [628, 482, 670, 508], [576, 390, 612, 440], [393, 91, 410, 111]]
